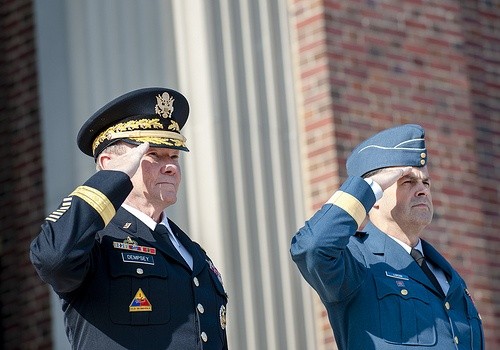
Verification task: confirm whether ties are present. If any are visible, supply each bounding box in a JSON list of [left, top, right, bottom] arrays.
[[156, 225, 189, 268], [411, 249, 446, 299]]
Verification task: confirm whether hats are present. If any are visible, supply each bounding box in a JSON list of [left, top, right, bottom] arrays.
[[77, 88, 190, 151], [345, 125, 427, 177]]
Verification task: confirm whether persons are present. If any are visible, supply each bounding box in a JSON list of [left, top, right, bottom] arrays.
[[289, 123, 485, 350], [30, 86, 229, 350]]
